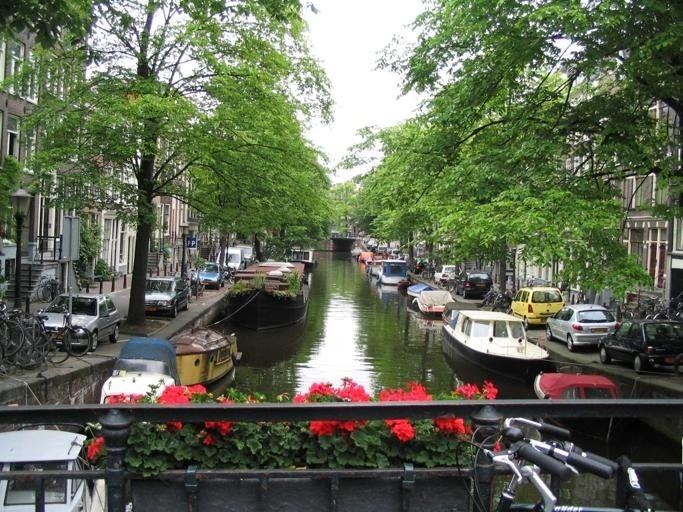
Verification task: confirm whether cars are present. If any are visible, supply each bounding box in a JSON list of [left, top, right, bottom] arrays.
[[434, 265, 462, 284], [409, 258, 427, 274], [455, 270, 494, 298], [145, 277, 190, 316], [597, 319, 683, 375], [545, 303, 620, 352], [39, 292, 121, 349]]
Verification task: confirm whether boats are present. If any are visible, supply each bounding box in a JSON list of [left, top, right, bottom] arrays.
[[0, 423, 107, 511], [288, 247, 315, 267], [169, 326, 243, 387], [348, 239, 411, 287], [226, 259, 311, 330], [406, 300, 419, 314], [376, 283, 405, 312], [408, 284, 431, 297], [414, 312, 449, 331], [223, 324, 307, 372], [93, 334, 180, 406], [200, 367, 237, 395], [414, 291, 453, 314], [441, 348, 550, 398], [533, 366, 617, 453], [442, 306, 550, 377]]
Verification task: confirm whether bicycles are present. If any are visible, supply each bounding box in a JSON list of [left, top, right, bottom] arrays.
[[619, 292, 683, 323], [421, 264, 434, 281], [479, 285, 515, 312], [1, 300, 93, 374], [440, 272, 457, 293], [460, 415, 672, 512]]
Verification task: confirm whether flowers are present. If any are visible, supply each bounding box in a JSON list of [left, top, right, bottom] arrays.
[[81, 378, 504, 476]]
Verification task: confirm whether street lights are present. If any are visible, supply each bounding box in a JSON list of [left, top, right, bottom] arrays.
[[178, 219, 189, 279], [9, 180, 37, 312]]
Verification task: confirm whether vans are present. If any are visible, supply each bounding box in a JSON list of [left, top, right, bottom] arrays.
[[511, 286, 566, 330], [189, 241, 279, 290]]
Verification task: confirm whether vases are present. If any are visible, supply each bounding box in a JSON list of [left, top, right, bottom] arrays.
[[86, 471, 493, 512]]
